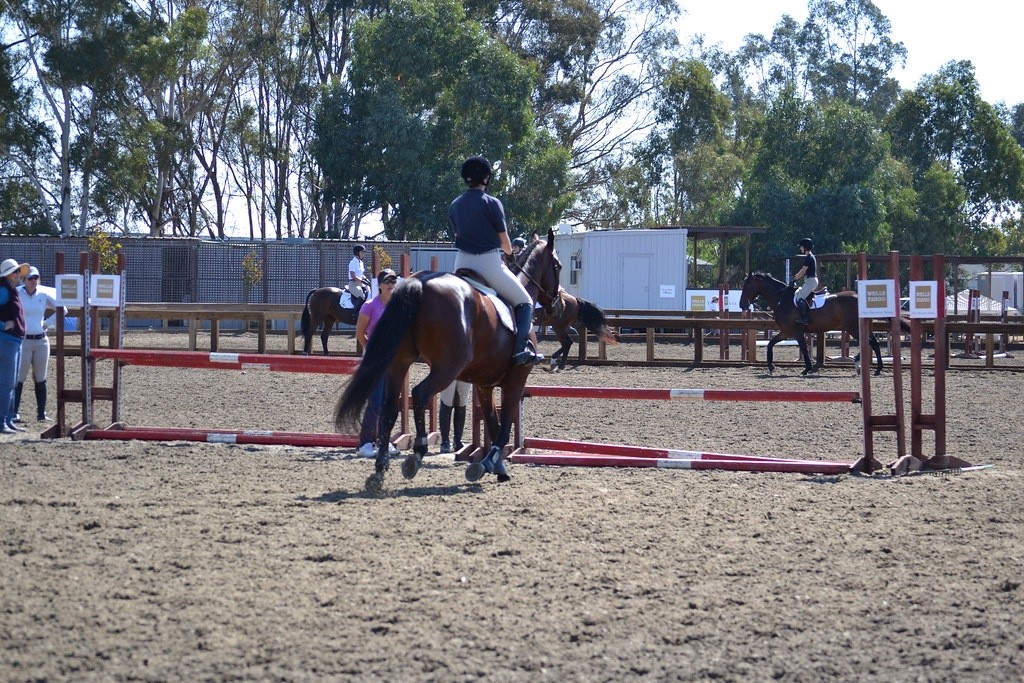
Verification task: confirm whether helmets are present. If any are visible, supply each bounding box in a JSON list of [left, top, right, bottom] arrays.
[[513, 238, 524, 248], [461, 157, 495, 184], [798, 237, 813, 248]]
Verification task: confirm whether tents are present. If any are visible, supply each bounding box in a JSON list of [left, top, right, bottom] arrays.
[[945, 289, 1021, 316]]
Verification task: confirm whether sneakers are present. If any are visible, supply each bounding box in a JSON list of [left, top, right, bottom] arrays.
[[375, 440, 401, 456], [358, 442, 379, 458]]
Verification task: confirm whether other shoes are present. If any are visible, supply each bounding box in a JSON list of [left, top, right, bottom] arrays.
[[0, 421, 16, 433], [452, 439, 463, 451], [37, 416, 45, 422], [7, 420, 25, 431], [512, 347, 545, 366], [352, 309, 359, 316], [440, 438, 450, 453], [11, 417, 20, 422]]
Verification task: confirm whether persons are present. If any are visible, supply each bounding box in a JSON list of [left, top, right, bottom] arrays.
[[502, 237, 538, 354], [12, 267, 68, 423], [0, 258, 30, 433], [710, 296, 721, 335], [348, 244, 371, 320], [449, 156, 546, 366], [356, 269, 401, 458], [789, 238, 819, 325], [439, 380, 471, 453]]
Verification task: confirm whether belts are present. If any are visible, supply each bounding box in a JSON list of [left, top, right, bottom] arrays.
[[19, 335, 24, 339], [26, 332, 45, 339]]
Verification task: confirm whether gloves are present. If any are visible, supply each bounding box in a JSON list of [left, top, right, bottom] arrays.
[[506, 253, 517, 269], [361, 278, 371, 286]]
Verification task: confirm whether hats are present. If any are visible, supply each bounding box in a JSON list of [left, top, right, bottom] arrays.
[[353, 244, 367, 255], [378, 268, 398, 284], [0, 258, 30, 277], [25, 266, 40, 278]]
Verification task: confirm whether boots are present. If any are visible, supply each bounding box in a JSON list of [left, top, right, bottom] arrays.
[[795, 298, 809, 325]]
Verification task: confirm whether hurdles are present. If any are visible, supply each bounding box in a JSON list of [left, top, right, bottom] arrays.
[[36, 250, 974, 476]]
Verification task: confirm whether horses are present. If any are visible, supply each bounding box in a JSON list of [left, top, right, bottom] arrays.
[[301, 266, 414, 357], [740, 270, 910, 377], [362, 227, 564, 488], [501, 251, 616, 373]]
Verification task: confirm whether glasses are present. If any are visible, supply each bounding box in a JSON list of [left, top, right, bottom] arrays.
[[14, 269, 21, 273], [28, 277, 37, 280], [383, 278, 397, 284]]
